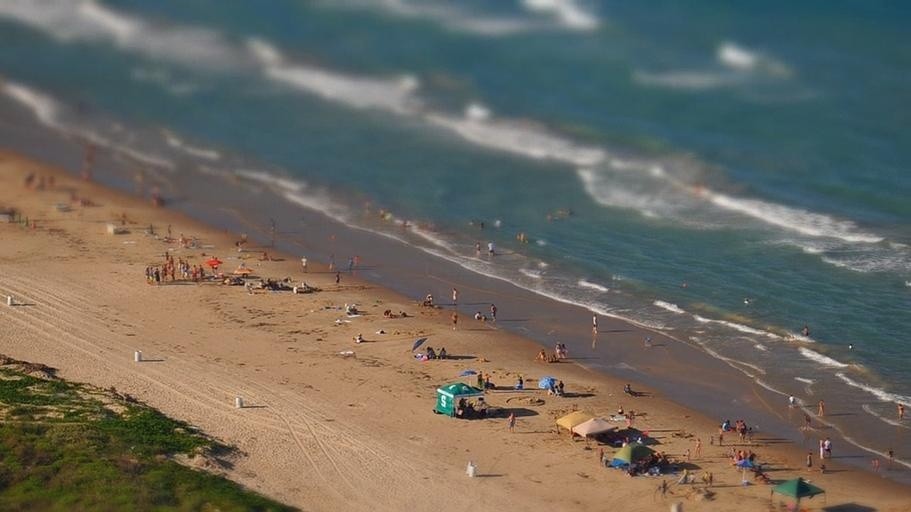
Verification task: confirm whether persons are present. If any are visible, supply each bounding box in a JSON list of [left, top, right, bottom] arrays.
[[122, 210, 904, 487]]
